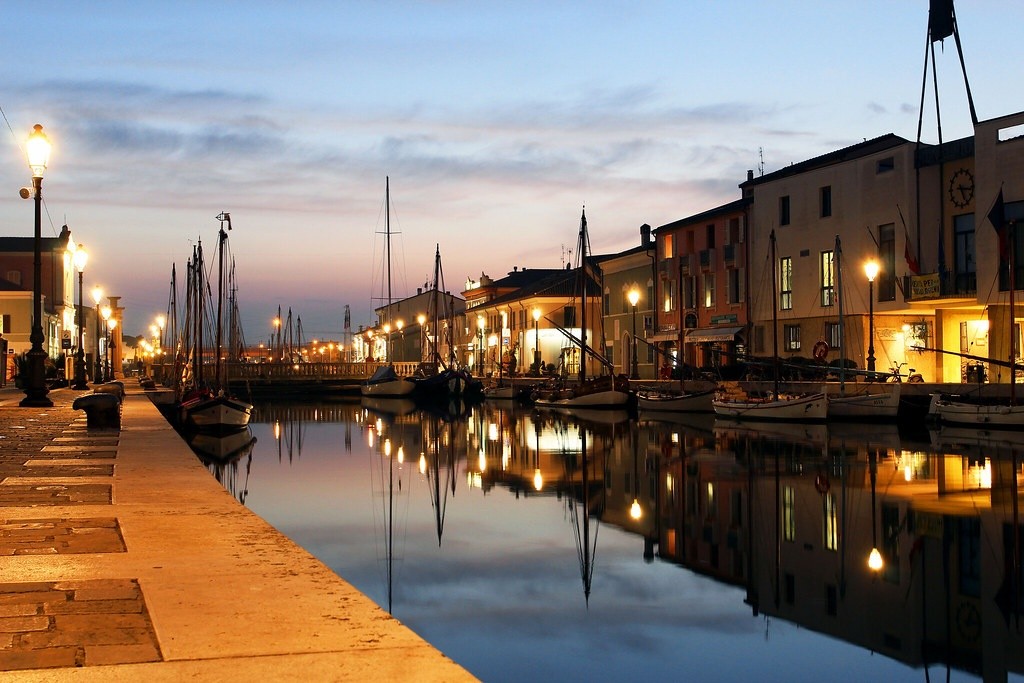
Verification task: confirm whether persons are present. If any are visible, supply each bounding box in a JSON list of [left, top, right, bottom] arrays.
[[137, 358, 143, 376]]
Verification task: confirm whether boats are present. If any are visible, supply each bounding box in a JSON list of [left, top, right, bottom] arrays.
[[896, 344, 1024, 443]]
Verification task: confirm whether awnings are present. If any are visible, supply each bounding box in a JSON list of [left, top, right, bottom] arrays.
[[653, 330, 680, 341], [685, 327, 743, 343]]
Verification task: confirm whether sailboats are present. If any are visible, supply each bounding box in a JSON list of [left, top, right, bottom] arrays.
[[633, 262, 727, 413], [480, 320, 520, 399], [402, 242, 486, 400], [782, 232, 902, 418], [711, 219, 830, 420], [533, 204, 631, 410], [934, 217, 1023, 443], [163, 210, 317, 432], [361, 174, 419, 414]]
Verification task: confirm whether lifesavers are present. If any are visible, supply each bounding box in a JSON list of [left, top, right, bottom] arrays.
[[180, 362, 189, 379], [813, 341, 829, 362]]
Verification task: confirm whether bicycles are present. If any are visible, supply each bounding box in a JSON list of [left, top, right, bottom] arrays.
[[888, 360, 924, 383]]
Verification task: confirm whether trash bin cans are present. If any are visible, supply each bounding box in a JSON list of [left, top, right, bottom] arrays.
[[966, 365, 984, 383]]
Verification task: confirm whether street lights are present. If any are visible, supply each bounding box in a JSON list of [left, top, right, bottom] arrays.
[[71, 243, 90, 390], [864, 262, 879, 382], [477, 318, 485, 378], [108, 319, 117, 381], [417, 315, 426, 362], [89, 285, 105, 384], [366, 331, 374, 362], [18, 122, 54, 408], [639, 222, 656, 347], [156, 315, 165, 364], [533, 310, 541, 372]]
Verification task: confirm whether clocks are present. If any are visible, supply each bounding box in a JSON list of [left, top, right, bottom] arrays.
[[945, 168, 975, 213]]
